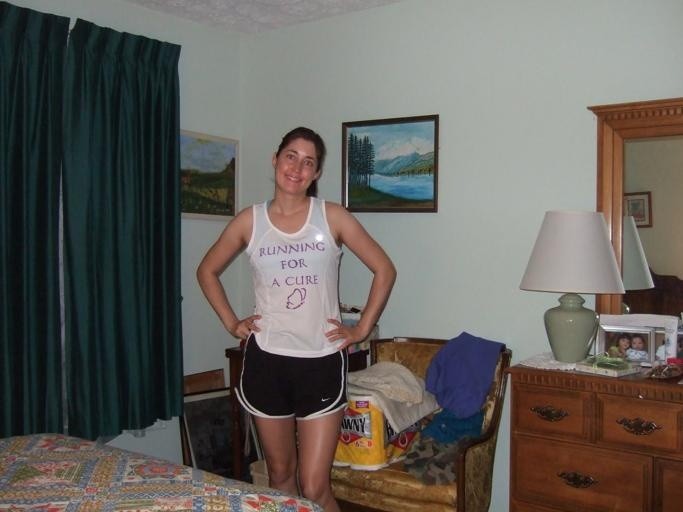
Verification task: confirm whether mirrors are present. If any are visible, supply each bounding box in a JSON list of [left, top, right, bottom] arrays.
[[586, 97, 683, 336]]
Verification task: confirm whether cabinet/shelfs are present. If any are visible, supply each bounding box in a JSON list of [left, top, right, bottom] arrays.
[[505, 352, 683, 512]]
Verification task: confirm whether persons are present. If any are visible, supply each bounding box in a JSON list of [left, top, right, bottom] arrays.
[[197, 128, 396, 512], [628, 335, 648, 362], [656, 339, 665, 358], [611, 334, 630, 359]]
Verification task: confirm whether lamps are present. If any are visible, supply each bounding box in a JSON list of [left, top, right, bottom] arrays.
[[622, 217, 655, 291], [520, 210, 627, 362]]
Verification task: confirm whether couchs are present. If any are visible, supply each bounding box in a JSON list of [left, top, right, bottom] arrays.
[[332, 336, 511, 511]]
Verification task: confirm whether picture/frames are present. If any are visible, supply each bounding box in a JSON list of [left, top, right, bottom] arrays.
[[179, 367, 264, 487], [623, 192, 653, 229], [181, 129, 240, 222], [341, 115, 440, 214], [599, 325, 656, 367], [598, 315, 678, 364]]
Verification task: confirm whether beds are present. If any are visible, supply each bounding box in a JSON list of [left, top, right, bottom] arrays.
[[2, 433, 324, 512]]
